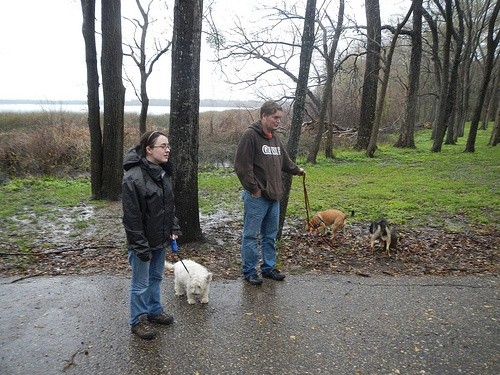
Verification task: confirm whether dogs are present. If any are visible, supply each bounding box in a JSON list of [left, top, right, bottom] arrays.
[[306, 209, 354, 240], [369, 219, 400, 255], [164, 259, 212, 304]]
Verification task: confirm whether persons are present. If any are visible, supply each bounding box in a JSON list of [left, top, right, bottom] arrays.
[[234, 101, 305, 285], [122, 130, 180, 339]]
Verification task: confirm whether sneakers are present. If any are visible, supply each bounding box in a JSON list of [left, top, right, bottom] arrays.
[[129, 312, 158, 340], [147, 306, 174, 325], [242, 271, 264, 286], [263, 267, 287, 281]]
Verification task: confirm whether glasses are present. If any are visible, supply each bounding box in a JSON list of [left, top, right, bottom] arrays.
[[147, 143, 172, 151]]
